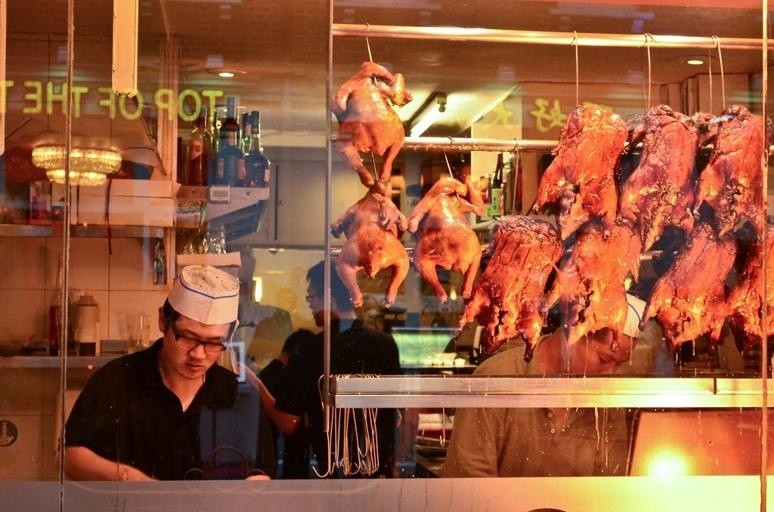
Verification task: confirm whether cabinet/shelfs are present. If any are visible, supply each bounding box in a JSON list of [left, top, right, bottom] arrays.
[[226, 147, 420, 248], [176, 185, 269, 273], [0, 223, 178, 369]]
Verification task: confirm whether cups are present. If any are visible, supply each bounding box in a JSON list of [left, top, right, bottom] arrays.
[[126, 312, 154, 354], [422, 357, 465, 367], [176, 220, 228, 255]]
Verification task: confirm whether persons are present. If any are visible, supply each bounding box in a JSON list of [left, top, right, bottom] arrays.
[[440, 301, 636, 480], [232, 257, 401, 478], [54, 264, 277, 485], [256, 328, 315, 475]]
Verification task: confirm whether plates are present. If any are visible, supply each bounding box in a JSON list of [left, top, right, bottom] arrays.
[[412, 435, 450, 459]]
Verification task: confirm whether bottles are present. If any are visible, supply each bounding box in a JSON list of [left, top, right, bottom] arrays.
[[75, 289, 100, 358], [185, 92, 271, 186], [474, 151, 519, 224], [48, 254, 73, 349]]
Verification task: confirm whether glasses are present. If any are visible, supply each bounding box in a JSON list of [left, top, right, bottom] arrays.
[[169, 322, 226, 352], [306, 294, 321, 303]]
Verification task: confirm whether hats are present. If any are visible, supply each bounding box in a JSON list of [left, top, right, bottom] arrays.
[[168, 264, 240, 326], [572, 286, 646, 338]]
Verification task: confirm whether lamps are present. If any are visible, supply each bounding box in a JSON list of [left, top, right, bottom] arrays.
[[401, 91, 447, 139], [29, 132, 122, 187]]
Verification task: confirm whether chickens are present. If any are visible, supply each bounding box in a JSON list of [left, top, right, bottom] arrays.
[[687, 104, 772, 244], [331, 61, 413, 188], [714, 200, 772, 355], [636, 198, 741, 356], [455, 214, 567, 365], [532, 105, 632, 230], [407, 175, 485, 304], [330, 179, 409, 310], [540, 213, 645, 355], [619, 104, 705, 253]]
